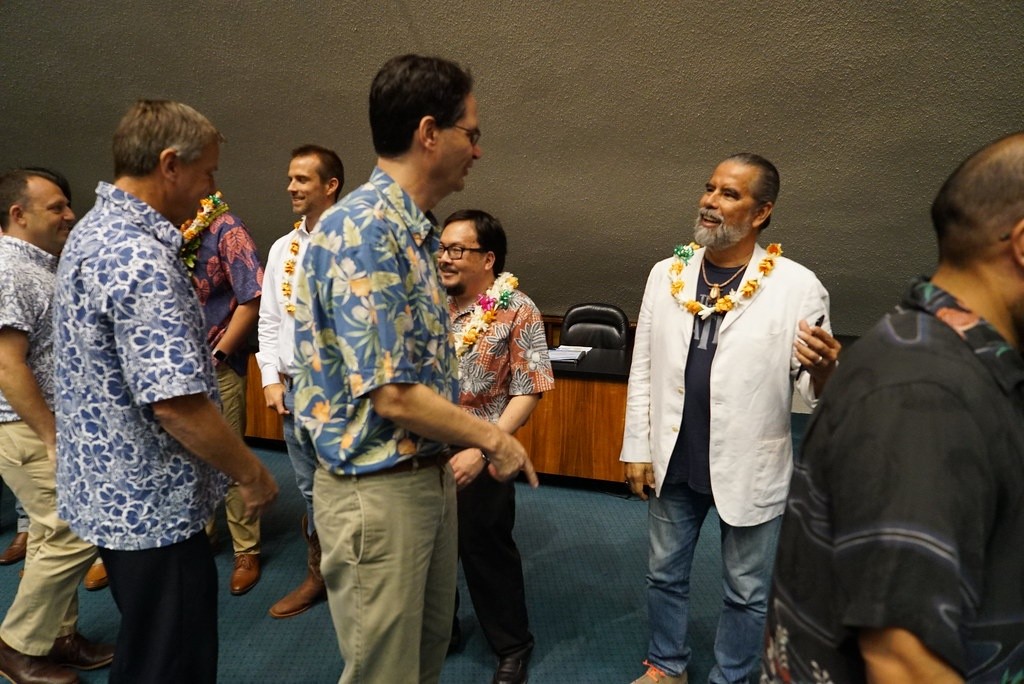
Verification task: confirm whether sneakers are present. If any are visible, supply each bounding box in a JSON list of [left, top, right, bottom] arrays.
[[631, 664, 689, 684]]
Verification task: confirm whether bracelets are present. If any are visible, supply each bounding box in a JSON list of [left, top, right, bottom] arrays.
[[479, 447, 492, 469]]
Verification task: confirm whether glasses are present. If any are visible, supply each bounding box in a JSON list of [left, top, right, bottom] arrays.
[[434, 242, 488, 261], [431, 116, 480, 147]]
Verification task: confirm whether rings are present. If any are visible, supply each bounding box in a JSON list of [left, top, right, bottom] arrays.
[[815, 356, 823, 365]]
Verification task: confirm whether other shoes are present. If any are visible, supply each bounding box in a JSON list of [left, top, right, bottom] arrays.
[[84, 563, 107, 591]]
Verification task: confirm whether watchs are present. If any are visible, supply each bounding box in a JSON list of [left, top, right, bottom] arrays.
[[210, 346, 228, 364]]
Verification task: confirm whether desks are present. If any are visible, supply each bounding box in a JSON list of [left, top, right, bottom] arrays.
[[244, 333, 864, 489]]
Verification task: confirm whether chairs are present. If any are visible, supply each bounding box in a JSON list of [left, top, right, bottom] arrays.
[[558, 303, 634, 347]]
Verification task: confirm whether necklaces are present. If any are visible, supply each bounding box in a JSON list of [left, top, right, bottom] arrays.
[[669, 242, 784, 319], [456, 271, 518, 359], [702, 257, 749, 301], [179, 195, 220, 246], [281, 221, 301, 317]]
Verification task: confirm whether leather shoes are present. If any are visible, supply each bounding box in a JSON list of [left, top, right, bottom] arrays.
[[0, 533, 27, 565], [231, 554, 261, 595], [494, 655, 530, 684], [0, 632, 115, 684]]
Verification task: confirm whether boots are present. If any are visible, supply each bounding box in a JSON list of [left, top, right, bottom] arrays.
[[268, 513, 328, 619]]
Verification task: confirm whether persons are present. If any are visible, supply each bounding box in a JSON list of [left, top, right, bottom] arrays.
[[52, 101, 278, 684], [178, 189, 265, 596], [618, 153, 842, 684], [0, 165, 115, 684], [255, 143, 346, 618], [291, 54, 539, 684], [765, 130, 1024, 684], [438, 208, 555, 684]]
